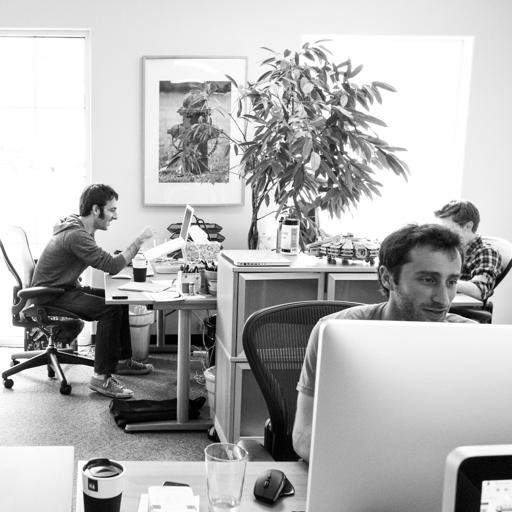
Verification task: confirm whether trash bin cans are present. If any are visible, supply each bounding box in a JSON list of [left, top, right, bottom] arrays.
[[203, 365, 216, 420], [128, 310, 154, 364]]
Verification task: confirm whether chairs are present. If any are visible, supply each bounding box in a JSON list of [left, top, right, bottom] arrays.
[[0, 224, 96, 398], [454, 236, 511, 323]]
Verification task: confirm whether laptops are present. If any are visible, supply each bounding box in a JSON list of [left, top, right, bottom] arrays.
[[143, 204, 194, 260], [220, 250, 292, 267]]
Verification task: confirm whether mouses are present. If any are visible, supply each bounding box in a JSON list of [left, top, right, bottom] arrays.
[[254, 469, 285, 504]]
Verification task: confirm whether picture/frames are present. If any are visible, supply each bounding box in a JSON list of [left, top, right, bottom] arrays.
[[141, 55, 249, 208]]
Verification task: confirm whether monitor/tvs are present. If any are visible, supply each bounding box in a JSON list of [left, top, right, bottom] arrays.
[[305, 320, 512, 512], [444, 447, 512, 512]]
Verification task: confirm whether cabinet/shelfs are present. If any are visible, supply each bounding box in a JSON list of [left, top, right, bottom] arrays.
[[216, 246, 390, 463]]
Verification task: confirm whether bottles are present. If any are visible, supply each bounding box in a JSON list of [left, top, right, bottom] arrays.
[[280, 218, 301, 256], [188, 281, 195, 296]]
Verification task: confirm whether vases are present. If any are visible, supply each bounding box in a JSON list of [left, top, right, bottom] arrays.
[[246, 215, 259, 250]]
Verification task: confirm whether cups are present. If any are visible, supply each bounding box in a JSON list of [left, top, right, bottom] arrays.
[[208, 280, 218, 296], [81, 457, 126, 512], [204, 442, 250, 512], [132, 258, 148, 283]]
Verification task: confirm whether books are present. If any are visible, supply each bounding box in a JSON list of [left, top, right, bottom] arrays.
[[112, 264, 176, 292]]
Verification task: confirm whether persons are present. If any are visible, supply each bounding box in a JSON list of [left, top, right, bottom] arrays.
[[435, 199, 500, 302], [291, 224, 482, 467], [30, 184, 157, 401]]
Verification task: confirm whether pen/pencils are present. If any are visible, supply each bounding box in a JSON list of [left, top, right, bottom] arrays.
[[180, 263, 199, 273], [201, 260, 217, 272], [112, 295, 128, 300]]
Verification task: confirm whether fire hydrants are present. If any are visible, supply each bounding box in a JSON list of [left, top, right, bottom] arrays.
[[166, 90, 223, 174]]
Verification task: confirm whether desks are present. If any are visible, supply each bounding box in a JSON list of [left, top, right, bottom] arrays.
[[101, 249, 216, 434], [0, 445, 76, 511], [76, 456, 315, 512]]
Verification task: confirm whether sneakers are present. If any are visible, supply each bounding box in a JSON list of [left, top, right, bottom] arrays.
[[116, 357, 154, 376], [88, 375, 134, 399]]
